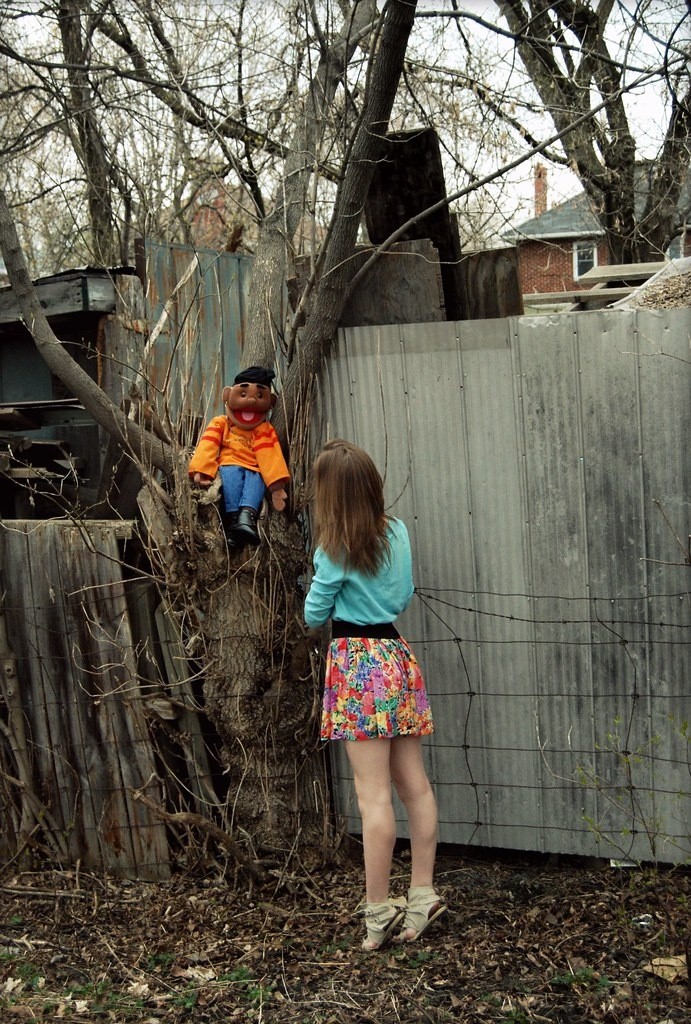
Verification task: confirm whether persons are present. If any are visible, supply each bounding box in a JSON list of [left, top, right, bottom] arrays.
[[305, 439, 448, 950], [188, 365, 290, 549]]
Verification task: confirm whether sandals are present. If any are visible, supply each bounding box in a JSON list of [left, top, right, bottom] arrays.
[[389, 886, 449, 947], [361, 902, 406, 952]]
